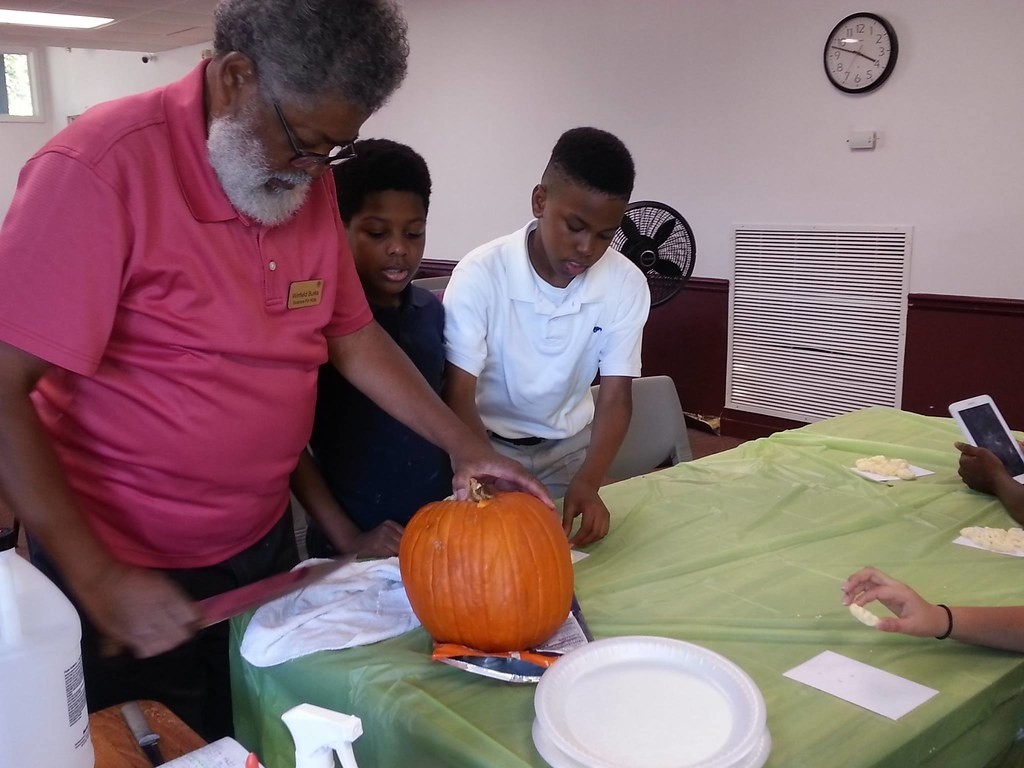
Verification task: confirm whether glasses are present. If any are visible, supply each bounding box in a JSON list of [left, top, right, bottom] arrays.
[[266, 83, 358, 169]]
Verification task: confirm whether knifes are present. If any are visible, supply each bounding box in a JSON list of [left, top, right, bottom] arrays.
[[95, 555, 358, 653], [120, 702, 175, 766]]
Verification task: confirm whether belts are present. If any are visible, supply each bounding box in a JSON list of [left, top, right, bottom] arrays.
[[487, 430, 545, 446]]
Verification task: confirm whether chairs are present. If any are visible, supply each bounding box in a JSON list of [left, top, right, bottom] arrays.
[[587, 376, 693, 486]]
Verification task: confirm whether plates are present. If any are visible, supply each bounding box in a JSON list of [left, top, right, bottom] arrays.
[[532, 636, 771, 768]]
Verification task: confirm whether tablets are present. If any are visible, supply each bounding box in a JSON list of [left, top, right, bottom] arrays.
[[949, 394, 1024, 485]]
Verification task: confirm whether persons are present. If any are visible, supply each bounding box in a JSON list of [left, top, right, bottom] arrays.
[[0, 0, 555, 743], [841, 565, 1024, 654], [442, 128, 651, 551], [289, 139, 454, 558], [954, 441, 1024, 525]]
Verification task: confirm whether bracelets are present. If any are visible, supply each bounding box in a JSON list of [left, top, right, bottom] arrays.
[[936, 605, 952, 640]]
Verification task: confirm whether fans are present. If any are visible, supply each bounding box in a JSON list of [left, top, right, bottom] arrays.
[[609, 200, 697, 309]]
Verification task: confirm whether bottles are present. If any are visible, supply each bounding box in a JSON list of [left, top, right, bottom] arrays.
[[1, 526, 96, 768]]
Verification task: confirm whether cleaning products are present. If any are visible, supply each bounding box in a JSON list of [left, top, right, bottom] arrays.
[[280, 703, 365, 768]]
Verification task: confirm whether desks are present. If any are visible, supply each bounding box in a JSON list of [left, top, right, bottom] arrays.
[[228, 405, 1024, 768]]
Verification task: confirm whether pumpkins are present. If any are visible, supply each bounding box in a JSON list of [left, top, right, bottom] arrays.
[[398, 478, 574, 656]]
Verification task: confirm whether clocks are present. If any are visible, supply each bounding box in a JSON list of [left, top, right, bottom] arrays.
[[822, 12, 899, 93]]
